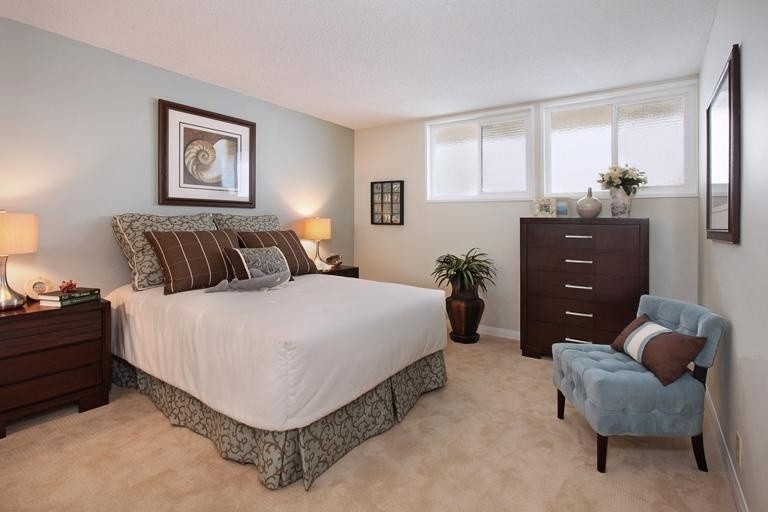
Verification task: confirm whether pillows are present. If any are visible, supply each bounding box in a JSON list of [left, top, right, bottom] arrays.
[[210, 210, 285, 234], [104, 205, 220, 292], [219, 244, 295, 291], [234, 227, 317, 278], [609, 311, 708, 387], [141, 226, 241, 296]]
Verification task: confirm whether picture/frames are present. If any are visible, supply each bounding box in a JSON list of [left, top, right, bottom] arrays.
[[151, 96, 257, 210], [702, 43, 743, 248], [531, 195, 557, 218], [556, 197, 572, 219], [368, 178, 406, 226]]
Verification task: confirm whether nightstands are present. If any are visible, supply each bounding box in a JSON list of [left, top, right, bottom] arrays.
[[315, 263, 360, 279], [1, 289, 114, 440]]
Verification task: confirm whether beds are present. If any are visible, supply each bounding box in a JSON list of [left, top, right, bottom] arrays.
[[96, 268, 452, 493]]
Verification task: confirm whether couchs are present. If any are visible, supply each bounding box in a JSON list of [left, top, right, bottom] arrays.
[[549, 290, 729, 478]]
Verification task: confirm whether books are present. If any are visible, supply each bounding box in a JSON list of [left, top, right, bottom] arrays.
[[38, 287, 101, 301], [37, 295, 100, 308]]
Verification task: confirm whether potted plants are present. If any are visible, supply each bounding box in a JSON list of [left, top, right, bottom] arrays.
[[429, 247, 499, 345]]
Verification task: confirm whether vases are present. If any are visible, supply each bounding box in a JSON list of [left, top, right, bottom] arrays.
[[606, 183, 635, 220], [574, 188, 602, 218]]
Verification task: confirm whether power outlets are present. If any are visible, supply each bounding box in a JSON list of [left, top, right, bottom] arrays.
[[733, 428, 744, 468]]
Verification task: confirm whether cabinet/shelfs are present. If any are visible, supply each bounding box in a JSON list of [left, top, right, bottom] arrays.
[[512, 210, 650, 363]]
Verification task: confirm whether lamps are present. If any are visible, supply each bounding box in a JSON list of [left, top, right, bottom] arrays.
[[300, 215, 335, 270], [0, 207, 41, 312]]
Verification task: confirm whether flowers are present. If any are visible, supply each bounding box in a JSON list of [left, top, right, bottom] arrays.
[[595, 162, 649, 197]]
